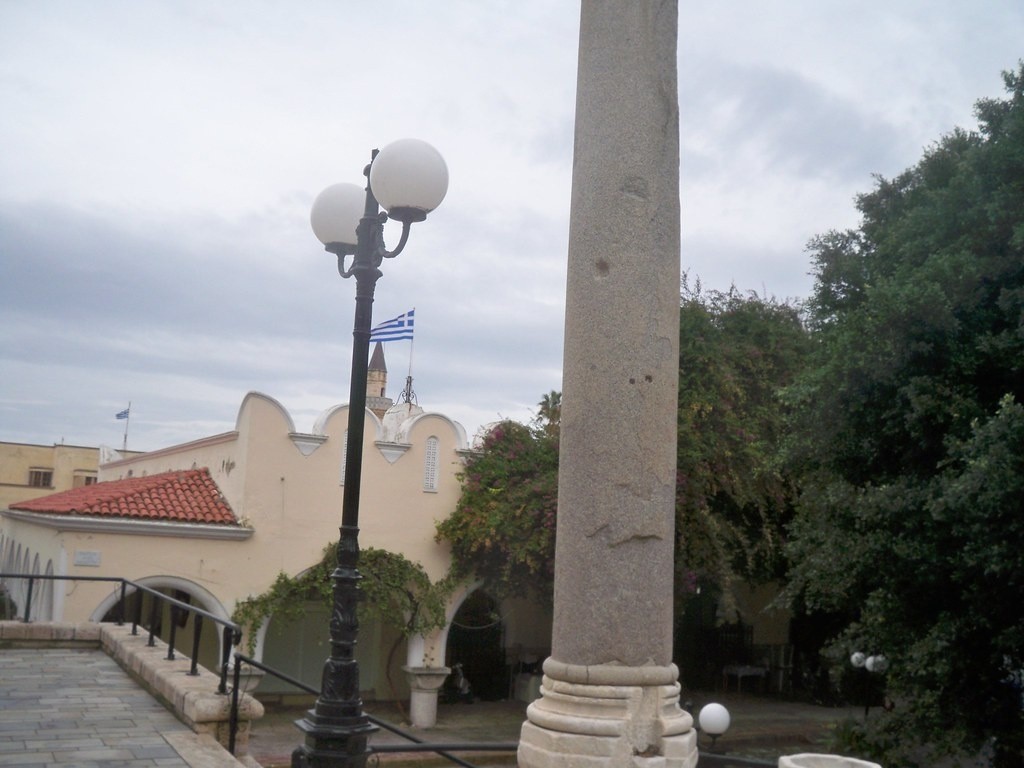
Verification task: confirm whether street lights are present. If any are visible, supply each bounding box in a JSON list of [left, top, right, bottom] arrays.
[[291, 138, 448, 768], [850, 652, 887, 722]]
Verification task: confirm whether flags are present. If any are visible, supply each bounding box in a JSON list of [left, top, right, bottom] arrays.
[[369, 311, 414, 342], [116, 409, 128, 419]]
